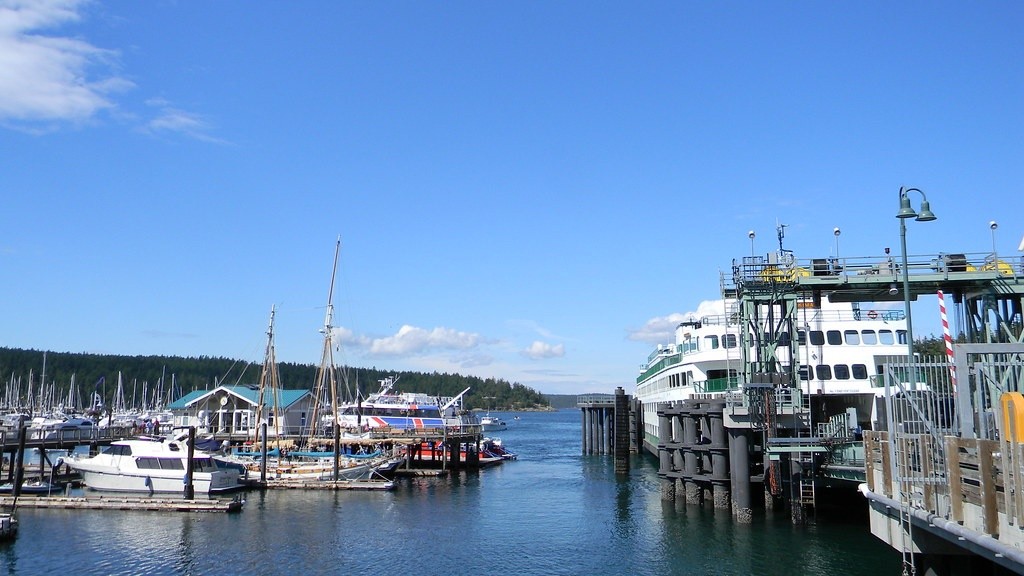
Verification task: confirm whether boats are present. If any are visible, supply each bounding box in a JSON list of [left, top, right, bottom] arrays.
[[633, 293, 928, 484]]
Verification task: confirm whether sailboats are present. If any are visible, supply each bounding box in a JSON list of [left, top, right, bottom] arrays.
[[0, 233, 519, 497]]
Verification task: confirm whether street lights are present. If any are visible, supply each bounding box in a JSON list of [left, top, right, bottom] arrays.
[[897, 185, 938, 475]]
